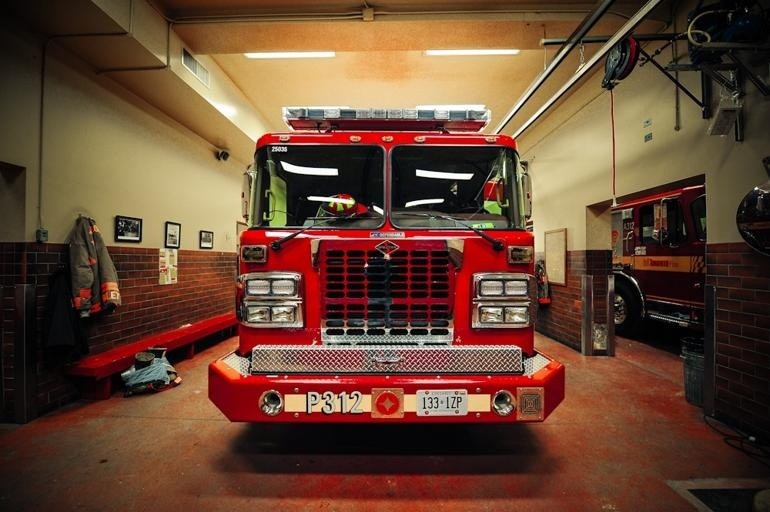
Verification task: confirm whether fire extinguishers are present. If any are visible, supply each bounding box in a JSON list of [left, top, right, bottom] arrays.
[[535, 259, 551, 304]]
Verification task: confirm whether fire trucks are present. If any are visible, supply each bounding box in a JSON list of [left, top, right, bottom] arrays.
[[208, 101, 566, 423], [611, 183, 708, 337]]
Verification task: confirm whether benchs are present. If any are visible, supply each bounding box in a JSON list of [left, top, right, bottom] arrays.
[[68, 308, 243, 400]]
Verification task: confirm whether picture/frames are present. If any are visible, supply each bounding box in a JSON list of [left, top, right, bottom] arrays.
[[200, 230, 214, 249], [165, 221, 181, 249], [114, 215, 143, 243], [542, 228, 566, 287]]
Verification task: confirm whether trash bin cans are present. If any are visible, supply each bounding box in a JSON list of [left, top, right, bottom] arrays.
[[680, 336, 706, 406]]
